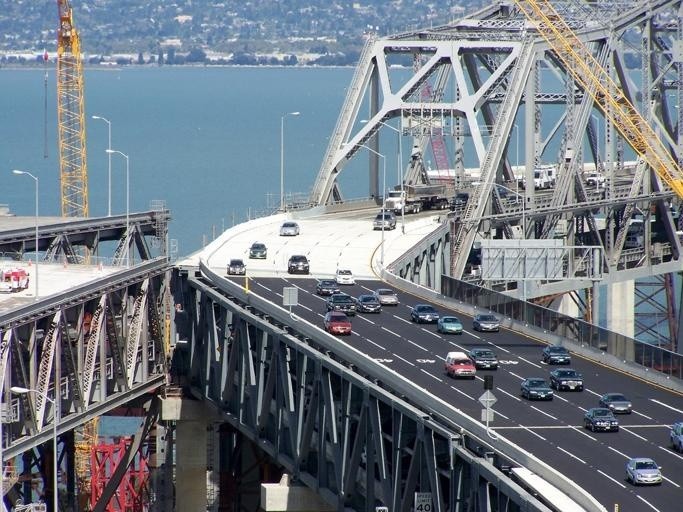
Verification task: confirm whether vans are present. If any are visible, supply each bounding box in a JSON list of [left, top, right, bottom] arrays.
[[624, 233, 644, 249]]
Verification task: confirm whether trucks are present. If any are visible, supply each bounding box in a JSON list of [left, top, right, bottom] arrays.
[[372, 163, 608, 224], [0, 264, 31, 295], [443, 351, 477, 380]]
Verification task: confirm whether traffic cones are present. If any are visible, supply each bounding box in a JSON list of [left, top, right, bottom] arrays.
[[27, 257, 33, 267], [96, 263, 105, 273], [62, 261, 68, 269]]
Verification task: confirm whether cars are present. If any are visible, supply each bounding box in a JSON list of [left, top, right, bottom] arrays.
[[519, 377, 555, 402], [436, 315, 464, 335], [315, 268, 439, 336], [278, 221, 300, 237], [623, 456, 663, 488], [360, 447, 375, 464], [669, 421, 682, 452], [248, 241, 268, 262], [541, 344, 571, 366], [472, 313, 499, 333], [548, 367, 584, 393], [597, 392, 633, 417], [225, 258, 247, 276]]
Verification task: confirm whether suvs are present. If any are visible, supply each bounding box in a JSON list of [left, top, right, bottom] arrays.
[[581, 408, 620, 435], [373, 210, 396, 230], [285, 255, 312, 276], [468, 347, 500, 371]]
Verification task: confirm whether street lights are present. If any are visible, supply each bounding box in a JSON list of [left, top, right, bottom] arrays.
[[338, 140, 386, 265], [104, 147, 130, 270], [469, 180, 527, 323], [358, 117, 408, 236], [277, 111, 302, 210], [11, 168, 40, 301], [90, 114, 113, 217]]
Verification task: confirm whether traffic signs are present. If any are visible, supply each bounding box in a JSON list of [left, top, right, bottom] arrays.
[[413, 491, 431, 512]]
